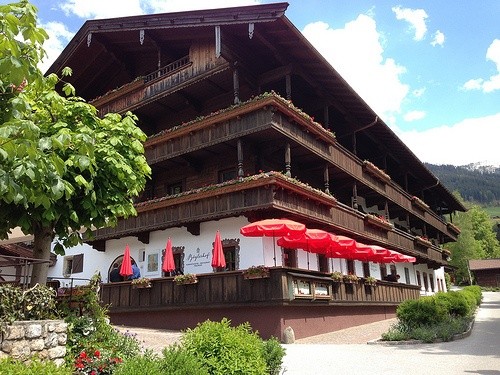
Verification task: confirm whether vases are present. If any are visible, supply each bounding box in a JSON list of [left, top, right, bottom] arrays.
[[446, 225, 461, 236], [416, 240, 431, 250], [145, 97, 336, 159], [132, 284, 151, 289], [333, 278, 343, 283], [245, 273, 270, 279], [365, 282, 377, 286], [413, 200, 429, 212], [367, 218, 392, 232], [178, 280, 198, 285], [345, 279, 359, 284], [134, 176, 334, 222], [363, 166, 388, 186], [92, 80, 146, 118]]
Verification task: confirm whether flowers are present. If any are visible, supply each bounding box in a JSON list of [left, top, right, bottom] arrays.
[[366, 214, 394, 229], [130, 171, 336, 208], [331, 271, 344, 279], [363, 159, 391, 181], [366, 277, 379, 283], [147, 89, 338, 139], [87, 76, 144, 104], [240, 265, 269, 276], [343, 274, 360, 281], [130, 278, 151, 287], [415, 236, 432, 247], [173, 275, 197, 284], [413, 195, 430, 210], [447, 221, 462, 234]]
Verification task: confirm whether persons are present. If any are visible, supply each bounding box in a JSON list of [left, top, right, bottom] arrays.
[[128, 262, 140, 280], [110, 263, 124, 282]]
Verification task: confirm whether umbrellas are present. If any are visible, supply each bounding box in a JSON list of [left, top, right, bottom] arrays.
[[163, 237, 176, 277], [120, 244, 133, 281], [211, 230, 226, 272], [240, 219, 416, 281]]
